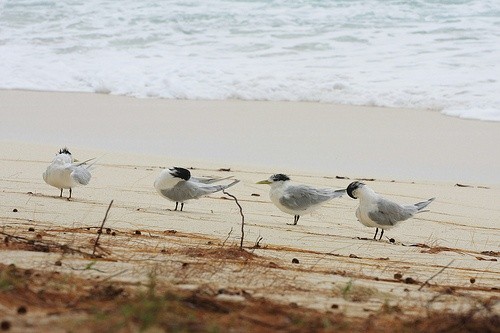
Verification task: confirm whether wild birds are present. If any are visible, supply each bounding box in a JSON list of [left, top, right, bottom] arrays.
[[42, 148, 97, 203], [256, 173, 347, 226], [334, 182, 436, 240], [152, 167, 240, 211]]
